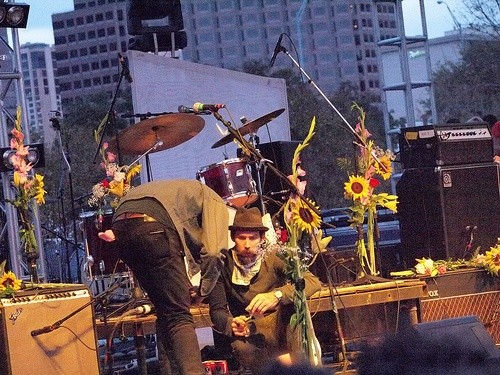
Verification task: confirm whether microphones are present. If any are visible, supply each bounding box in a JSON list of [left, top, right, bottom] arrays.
[[118, 52, 133, 83], [178, 105, 212, 115], [193, 102, 226, 112], [269, 34, 283, 67], [31, 325, 52, 336]]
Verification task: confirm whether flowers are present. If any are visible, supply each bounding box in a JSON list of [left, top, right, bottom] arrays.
[[0, 259, 23, 302], [9, 104, 49, 254], [336, 101, 401, 279], [408, 244, 500, 276], [242, 115, 333, 371], [86, 112, 142, 213]]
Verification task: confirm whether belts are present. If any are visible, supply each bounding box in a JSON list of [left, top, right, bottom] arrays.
[[115, 212, 146, 222]]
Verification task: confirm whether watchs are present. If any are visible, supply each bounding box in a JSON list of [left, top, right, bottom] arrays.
[[274, 291, 282, 300]]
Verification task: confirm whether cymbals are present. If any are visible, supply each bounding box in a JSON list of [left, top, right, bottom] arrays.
[[211, 107, 285, 149], [108, 114, 205, 156]]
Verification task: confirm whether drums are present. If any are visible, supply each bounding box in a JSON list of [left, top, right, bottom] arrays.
[[195, 157, 259, 210], [80, 209, 133, 283]]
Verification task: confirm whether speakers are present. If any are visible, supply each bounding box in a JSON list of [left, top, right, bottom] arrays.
[[395, 163, 500, 268], [362, 315, 500, 375], [416, 272, 500, 344], [236, 141, 308, 230], [0, 284, 100, 375]]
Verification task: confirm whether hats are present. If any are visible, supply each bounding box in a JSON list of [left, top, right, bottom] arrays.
[[228, 206, 269, 232]]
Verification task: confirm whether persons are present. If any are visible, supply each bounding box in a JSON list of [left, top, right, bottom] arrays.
[[113, 178, 230, 375], [447, 114, 500, 178], [201, 205, 321, 375]]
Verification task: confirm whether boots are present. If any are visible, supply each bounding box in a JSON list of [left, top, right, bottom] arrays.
[[169, 320, 211, 375], [156, 316, 180, 375]]
